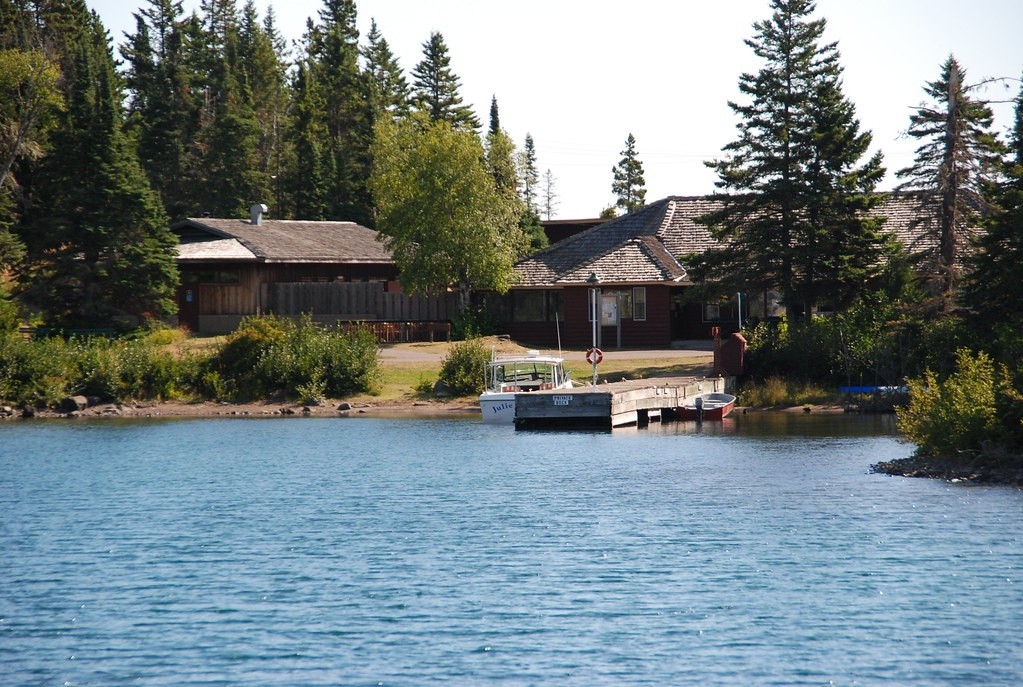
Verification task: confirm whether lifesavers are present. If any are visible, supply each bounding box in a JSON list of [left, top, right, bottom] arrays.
[[586, 348, 603, 365]]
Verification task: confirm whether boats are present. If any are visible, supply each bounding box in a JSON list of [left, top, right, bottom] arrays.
[[678, 392, 737, 420], [479, 312, 574, 423]]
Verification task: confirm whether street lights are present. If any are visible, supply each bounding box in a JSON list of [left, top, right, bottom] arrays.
[[585, 273, 601, 389]]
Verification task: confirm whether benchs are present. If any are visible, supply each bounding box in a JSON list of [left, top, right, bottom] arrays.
[[705, 400, 721, 402], [704, 403, 728, 405]]
